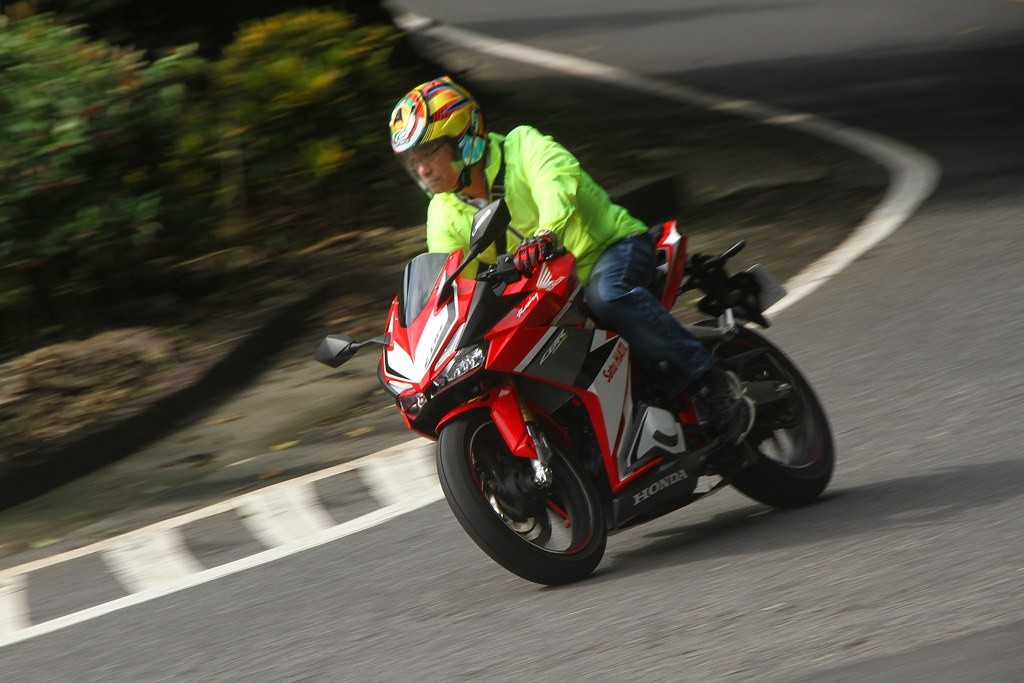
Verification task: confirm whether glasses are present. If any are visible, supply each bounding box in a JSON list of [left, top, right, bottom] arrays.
[[404, 141, 451, 170]]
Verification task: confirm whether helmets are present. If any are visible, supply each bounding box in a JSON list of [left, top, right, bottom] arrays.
[[389, 74, 488, 166]]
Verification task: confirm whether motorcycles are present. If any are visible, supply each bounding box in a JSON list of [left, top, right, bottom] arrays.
[[312, 193, 838, 586]]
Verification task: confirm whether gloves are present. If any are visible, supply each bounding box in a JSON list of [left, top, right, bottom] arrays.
[[512, 231, 555, 281]]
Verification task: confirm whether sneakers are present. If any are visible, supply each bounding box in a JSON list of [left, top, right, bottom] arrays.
[[696, 366, 755, 448]]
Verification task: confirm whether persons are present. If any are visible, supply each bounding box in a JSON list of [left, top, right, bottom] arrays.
[[390, 75, 736, 427]]
